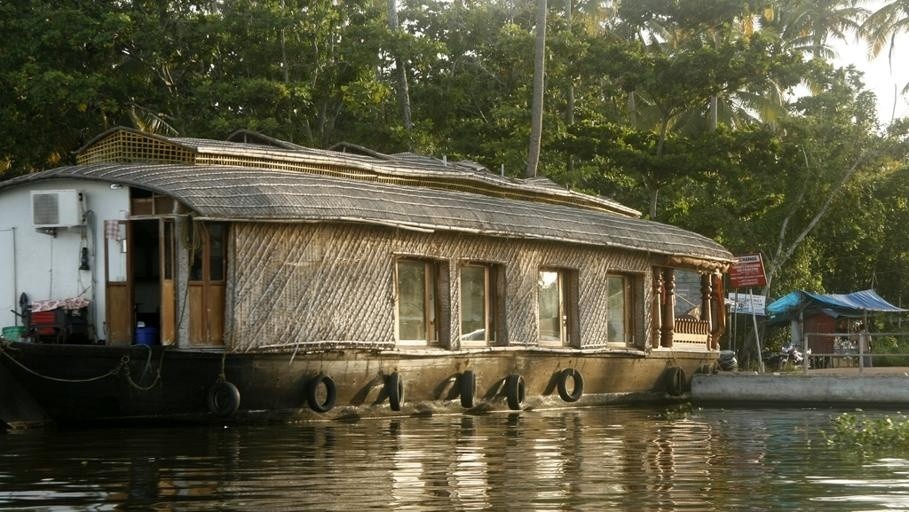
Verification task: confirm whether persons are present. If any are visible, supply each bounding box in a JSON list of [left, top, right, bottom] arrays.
[[858, 324, 872, 367]]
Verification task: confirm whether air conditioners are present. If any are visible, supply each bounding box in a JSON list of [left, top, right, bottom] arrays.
[[29, 189, 89, 234]]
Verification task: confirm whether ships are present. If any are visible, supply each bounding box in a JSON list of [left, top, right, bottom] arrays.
[[1, 126, 738, 419]]
